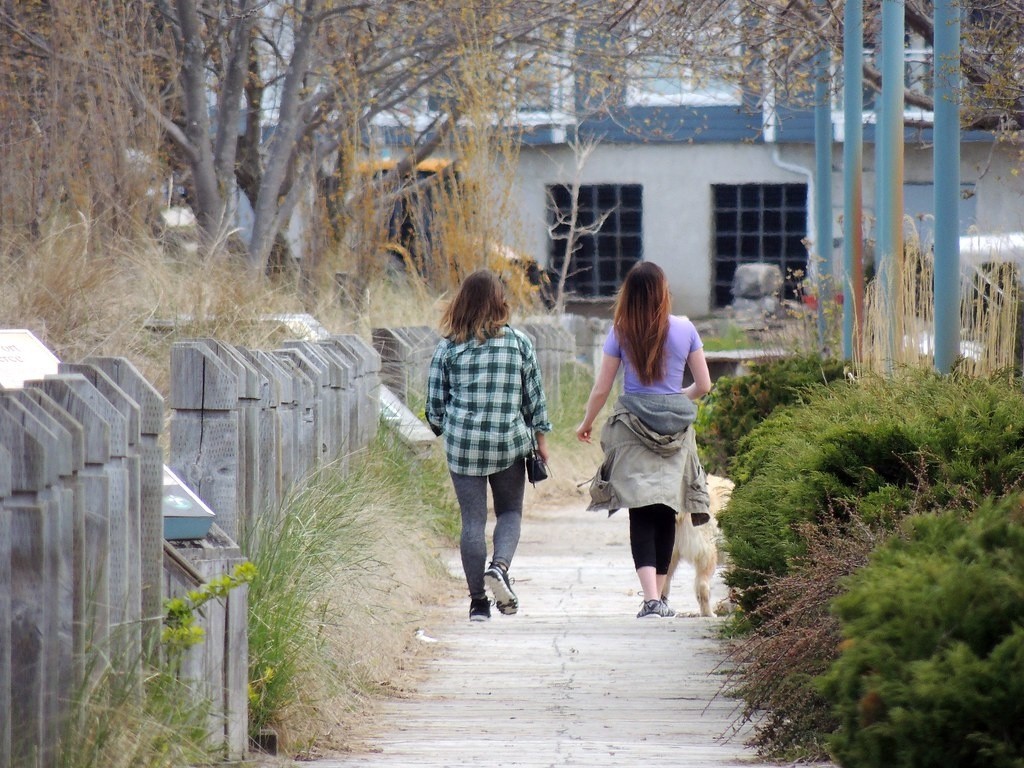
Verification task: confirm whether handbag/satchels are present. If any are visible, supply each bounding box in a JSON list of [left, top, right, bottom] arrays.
[[526, 449, 548, 484]]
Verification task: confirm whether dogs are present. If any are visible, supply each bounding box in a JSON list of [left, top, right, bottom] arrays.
[[663, 473, 736, 618]]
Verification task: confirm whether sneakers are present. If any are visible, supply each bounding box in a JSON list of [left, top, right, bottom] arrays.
[[634, 599, 662, 618], [483, 561, 519, 615], [659, 598, 678, 619], [468, 595, 492, 621]]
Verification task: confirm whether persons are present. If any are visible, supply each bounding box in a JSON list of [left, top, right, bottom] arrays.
[[424, 267, 552, 621], [578, 262, 710, 618]]
